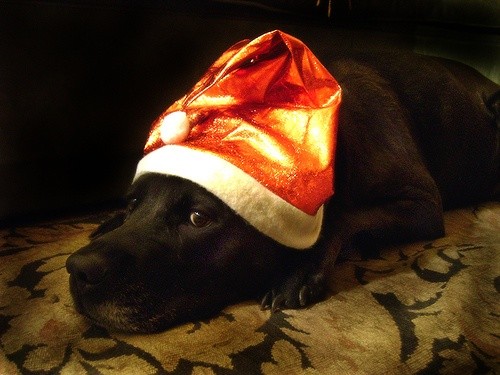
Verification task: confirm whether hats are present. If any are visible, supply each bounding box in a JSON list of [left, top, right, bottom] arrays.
[[131, 30, 344, 250]]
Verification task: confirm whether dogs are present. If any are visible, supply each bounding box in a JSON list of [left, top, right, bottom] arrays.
[[65, 30, 500, 335]]
